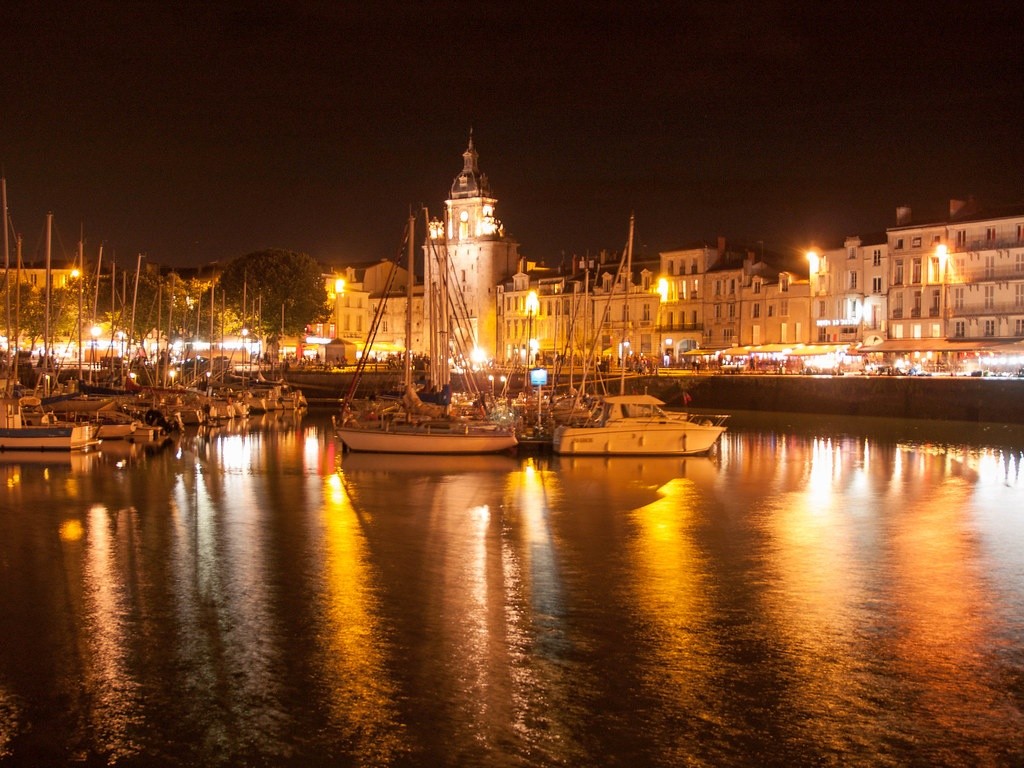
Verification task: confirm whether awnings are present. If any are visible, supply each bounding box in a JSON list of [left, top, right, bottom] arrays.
[[681, 337, 1024, 356]]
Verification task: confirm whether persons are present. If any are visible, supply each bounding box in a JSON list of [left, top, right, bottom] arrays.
[[535, 348, 845, 375]]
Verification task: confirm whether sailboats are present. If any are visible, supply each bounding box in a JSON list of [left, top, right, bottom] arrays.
[[332, 205, 731, 455], [0, 177, 308, 448]]
